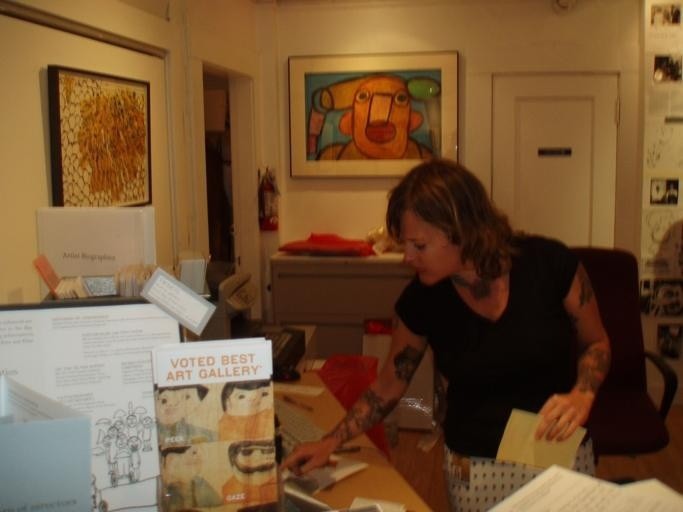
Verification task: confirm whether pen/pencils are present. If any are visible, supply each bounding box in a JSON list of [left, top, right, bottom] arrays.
[[333, 447, 361, 453], [284, 394, 312, 412]]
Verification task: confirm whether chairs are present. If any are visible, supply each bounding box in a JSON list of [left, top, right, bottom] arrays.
[[575, 246, 678, 464]]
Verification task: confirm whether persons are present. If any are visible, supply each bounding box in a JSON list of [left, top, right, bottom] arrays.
[[268, 157, 618, 511], [651, 186, 663, 203], [665, 183, 677, 204]]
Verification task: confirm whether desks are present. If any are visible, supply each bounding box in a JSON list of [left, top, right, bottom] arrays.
[[275, 372, 434, 512], [269, 250, 419, 335]]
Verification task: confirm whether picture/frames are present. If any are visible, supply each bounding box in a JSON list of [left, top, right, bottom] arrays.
[[287, 49, 464, 180], [47, 63, 153, 214]]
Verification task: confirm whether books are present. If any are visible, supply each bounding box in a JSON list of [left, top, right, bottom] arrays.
[[51, 261, 156, 300]]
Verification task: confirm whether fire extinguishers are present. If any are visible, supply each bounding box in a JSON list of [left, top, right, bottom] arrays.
[[258, 168, 280, 231]]
